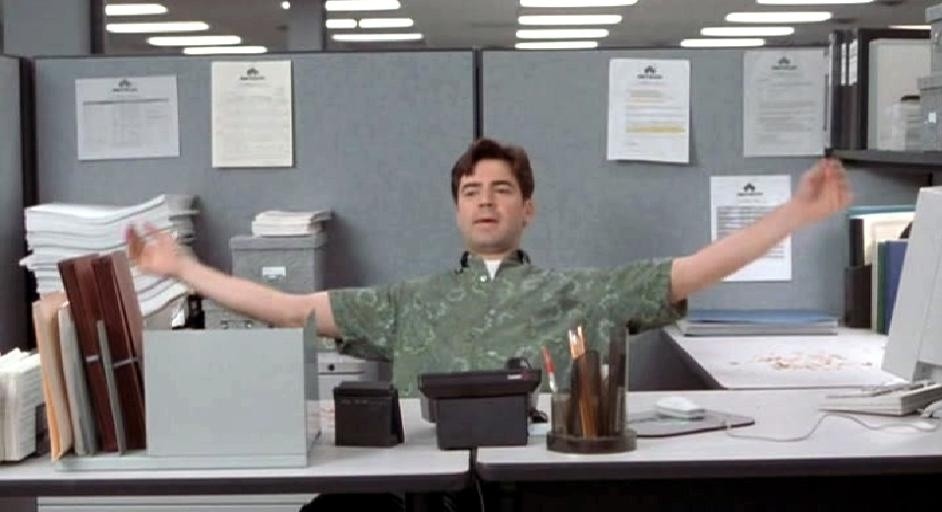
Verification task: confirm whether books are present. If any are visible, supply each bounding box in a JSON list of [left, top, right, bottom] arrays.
[[251, 209, 333, 236], [875, 238, 906, 339]]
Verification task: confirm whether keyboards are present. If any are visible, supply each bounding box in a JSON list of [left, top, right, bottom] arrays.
[[820, 378, 942, 416]]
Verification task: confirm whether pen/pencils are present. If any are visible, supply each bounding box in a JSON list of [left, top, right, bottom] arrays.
[[541, 346, 567, 432], [568, 324, 628, 440]]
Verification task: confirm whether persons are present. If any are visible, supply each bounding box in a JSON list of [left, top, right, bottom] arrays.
[[123, 142, 855, 512]]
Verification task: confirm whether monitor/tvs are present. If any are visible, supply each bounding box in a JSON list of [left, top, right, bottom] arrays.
[[881, 185, 942, 388]]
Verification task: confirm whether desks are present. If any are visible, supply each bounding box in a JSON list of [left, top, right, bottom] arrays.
[[659, 319, 907, 390], [475, 389, 942, 512], [0, 397, 473, 510]]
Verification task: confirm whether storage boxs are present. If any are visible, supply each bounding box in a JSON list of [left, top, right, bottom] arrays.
[[230, 232, 329, 296], [317, 351, 379, 400], [925, 4, 942, 73], [202, 299, 318, 402], [916, 74, 941, 152]]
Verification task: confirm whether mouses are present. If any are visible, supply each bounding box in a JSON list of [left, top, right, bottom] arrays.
[[655, 397, 705, 419]]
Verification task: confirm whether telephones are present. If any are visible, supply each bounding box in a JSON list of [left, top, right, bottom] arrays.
[[506, 357, 538, 416]]
